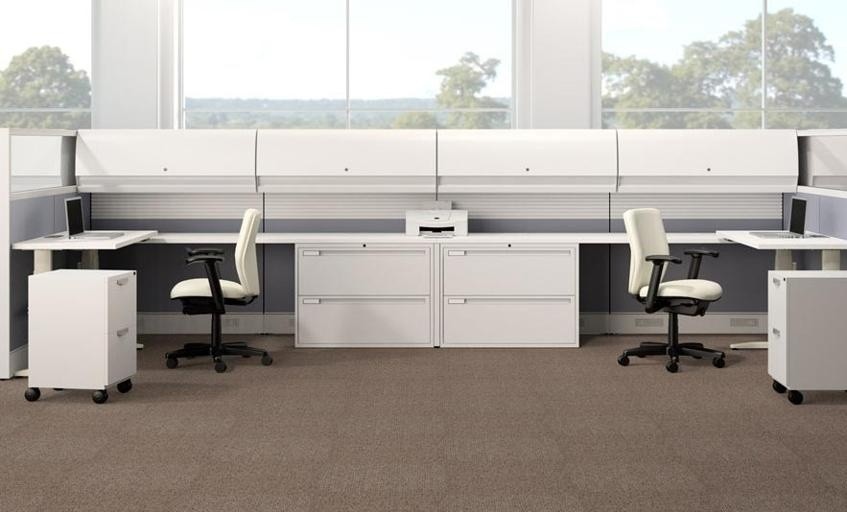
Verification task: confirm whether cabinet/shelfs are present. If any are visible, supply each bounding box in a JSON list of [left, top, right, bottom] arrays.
[[760, 270, 847, 407], [25, 268, 138, 405]]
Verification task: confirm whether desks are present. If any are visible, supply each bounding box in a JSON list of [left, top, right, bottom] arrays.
[[10, 228, 847, 376]]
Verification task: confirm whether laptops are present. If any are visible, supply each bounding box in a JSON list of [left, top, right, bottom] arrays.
[[749, 194, 808, 241], [63, 197, 124, 239]]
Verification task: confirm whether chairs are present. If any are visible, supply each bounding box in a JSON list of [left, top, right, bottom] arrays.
[[618, 202, 729, 372], [166, 207, 273, 374]]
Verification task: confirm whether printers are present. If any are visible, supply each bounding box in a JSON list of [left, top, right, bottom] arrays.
[[404, 208, 471, 239]]
